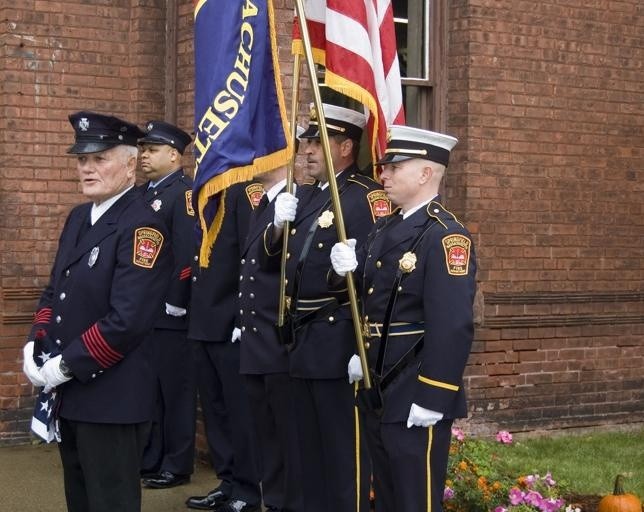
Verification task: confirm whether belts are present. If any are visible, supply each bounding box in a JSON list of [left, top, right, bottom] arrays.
[[366, 321, 427, 336], [285, 297, 361, 312]]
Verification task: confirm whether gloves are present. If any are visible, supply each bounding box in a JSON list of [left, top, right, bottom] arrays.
[[232, 327, 242, 344], [24, 341, 48, 388], [39, 353, 74, 395], [407, 403, 444, 430], [274, 182, 299, 231], [330, 238, 358, 279], [348, 355, 363, 385], [166, 302, 188, 317]]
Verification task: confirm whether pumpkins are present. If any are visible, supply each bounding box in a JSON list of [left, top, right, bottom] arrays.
[[598, 475, 642, 511]]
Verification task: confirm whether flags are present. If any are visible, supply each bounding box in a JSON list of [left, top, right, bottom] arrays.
[[191, 0, 294, 270], [291, 0, 410, 187]]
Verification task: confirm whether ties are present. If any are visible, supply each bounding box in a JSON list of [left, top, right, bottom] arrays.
[[256, 194, 270, 222]]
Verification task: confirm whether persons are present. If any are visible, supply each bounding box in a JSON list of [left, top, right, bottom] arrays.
[[238, 120, 308, 512], [22, 111, 175, 512], [330, 123, 478, 512], [134, 116, 196, 488], [258, 101, 396, 512], [183, 120, 306, 512]]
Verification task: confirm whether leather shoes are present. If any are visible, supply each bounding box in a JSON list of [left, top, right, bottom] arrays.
[[143, 471, 192, 489], [214, 497, 263, 512], [186, 488, 230, 511]]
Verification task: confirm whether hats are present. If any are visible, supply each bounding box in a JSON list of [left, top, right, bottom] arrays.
[[67, 113, 149, 155], [139, 120, 192, 156], [374, 125, 459, 167], [289, 121, 307, 143], [299, 102, 368, 142]]
